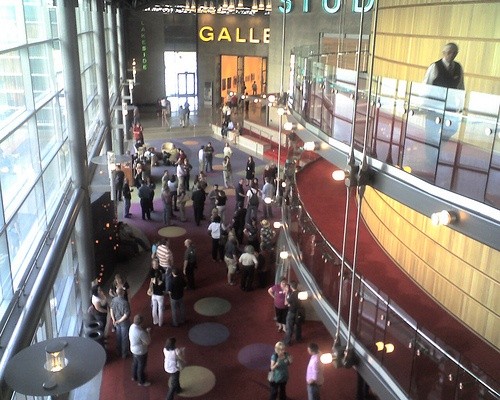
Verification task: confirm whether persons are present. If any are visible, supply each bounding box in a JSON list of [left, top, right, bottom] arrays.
[[91, 274, 130, 361], [223, 156, 233, 188], [231, 122, 240, 145], [223, 143, 232, 158], [252, 82, 257, 95], [133, 122, 144, 143], [178, 101, 190, 128], [146, 239, 196, 329], [162, 337, 185, 400], [269, 341, 293, 400], [221, 105, 232, 138], [205, 142, 214, 172], [246, 156, 255, 186], [268, 277, 304, 345], [199, 145, 206, 172], [305, 344, 324, 400], [115, 141, 208, 227], [423, 43, 466, 167], [128, 314, 152, 387], [259, 163, 277, 184], [208, 177, 275, 293]]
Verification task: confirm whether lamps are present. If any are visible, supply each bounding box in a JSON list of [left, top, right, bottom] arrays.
[[203, 0, 221, 14], [222, 0, 244, 12], [251, 0, 272, 13], [4, 336, 107, 400], [185, 0, 202, 14]]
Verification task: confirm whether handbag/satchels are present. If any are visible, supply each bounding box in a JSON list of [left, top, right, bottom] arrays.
[[266, 370, 277, 381], [147, 278, 154, 296], [175, 349, 183, 370]]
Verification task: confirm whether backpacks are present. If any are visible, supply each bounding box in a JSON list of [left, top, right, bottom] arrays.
[[249, 188, 259, 209]]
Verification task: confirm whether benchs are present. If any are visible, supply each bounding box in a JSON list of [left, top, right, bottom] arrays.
[[211, 119, 272, 155]]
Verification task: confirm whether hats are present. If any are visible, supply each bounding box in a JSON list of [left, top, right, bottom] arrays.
[[260, 220, 270, 228], [244, 245, 253, 253]]
